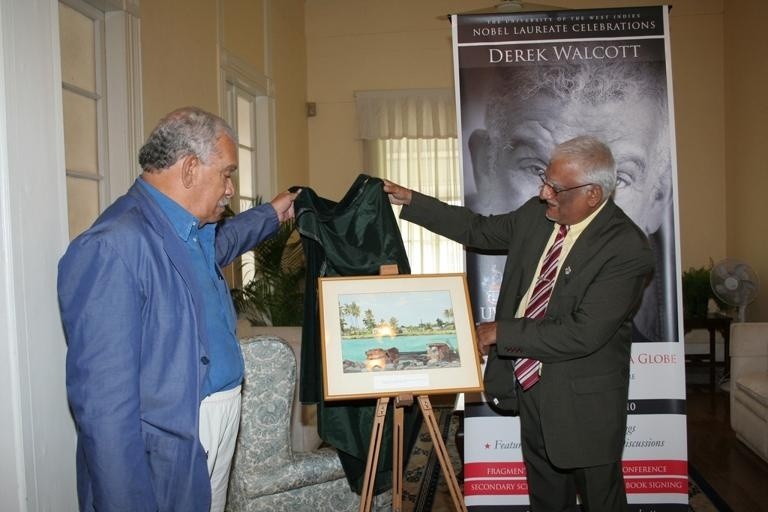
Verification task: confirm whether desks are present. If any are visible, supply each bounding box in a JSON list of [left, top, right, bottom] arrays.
[[685, 312, 733, 378]]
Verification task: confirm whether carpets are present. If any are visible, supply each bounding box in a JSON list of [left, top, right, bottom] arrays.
[[402, 406, 732, 512]]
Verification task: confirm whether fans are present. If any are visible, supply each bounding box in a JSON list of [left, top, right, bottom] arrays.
[[709, 257, 760, 392]]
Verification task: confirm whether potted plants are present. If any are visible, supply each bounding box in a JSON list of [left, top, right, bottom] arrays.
[[682, 266, 733, 319]]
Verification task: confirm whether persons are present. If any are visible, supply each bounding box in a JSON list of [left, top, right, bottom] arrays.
[[56, 107, 301, 512], [383, 135, 655, 510], [467, 46, 673, 344]]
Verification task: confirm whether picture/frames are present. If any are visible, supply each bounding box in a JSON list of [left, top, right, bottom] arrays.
[[317, 273, 486, 402]]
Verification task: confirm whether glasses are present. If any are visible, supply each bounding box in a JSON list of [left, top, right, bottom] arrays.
[[539, 171, 595, 198]]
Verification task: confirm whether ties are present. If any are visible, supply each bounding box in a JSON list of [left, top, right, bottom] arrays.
[[515, 223, 570, 392]]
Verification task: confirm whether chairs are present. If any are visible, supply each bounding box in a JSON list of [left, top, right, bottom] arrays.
[[226, 336, 392, 512], [729, 322, 768, 464]]
